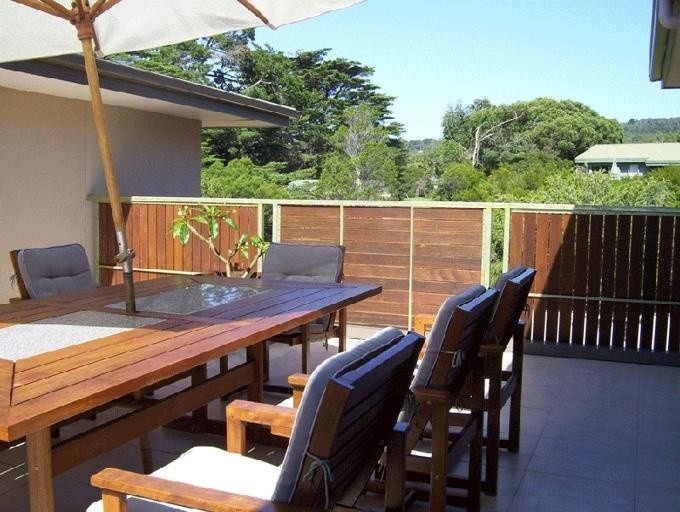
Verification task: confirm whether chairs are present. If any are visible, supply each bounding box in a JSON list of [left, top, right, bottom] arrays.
[[9, 243, 102, 300], [261, 242, 350, 382], [272, 284, 501, 511], [414, 261, 535, 498], [84, 325, 426, 512]]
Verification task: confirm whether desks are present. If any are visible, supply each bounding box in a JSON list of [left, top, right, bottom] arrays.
[[1, 274, 386, 511]]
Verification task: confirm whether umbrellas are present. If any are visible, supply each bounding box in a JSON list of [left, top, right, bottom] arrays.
[[1, 0, 372, 316]]
